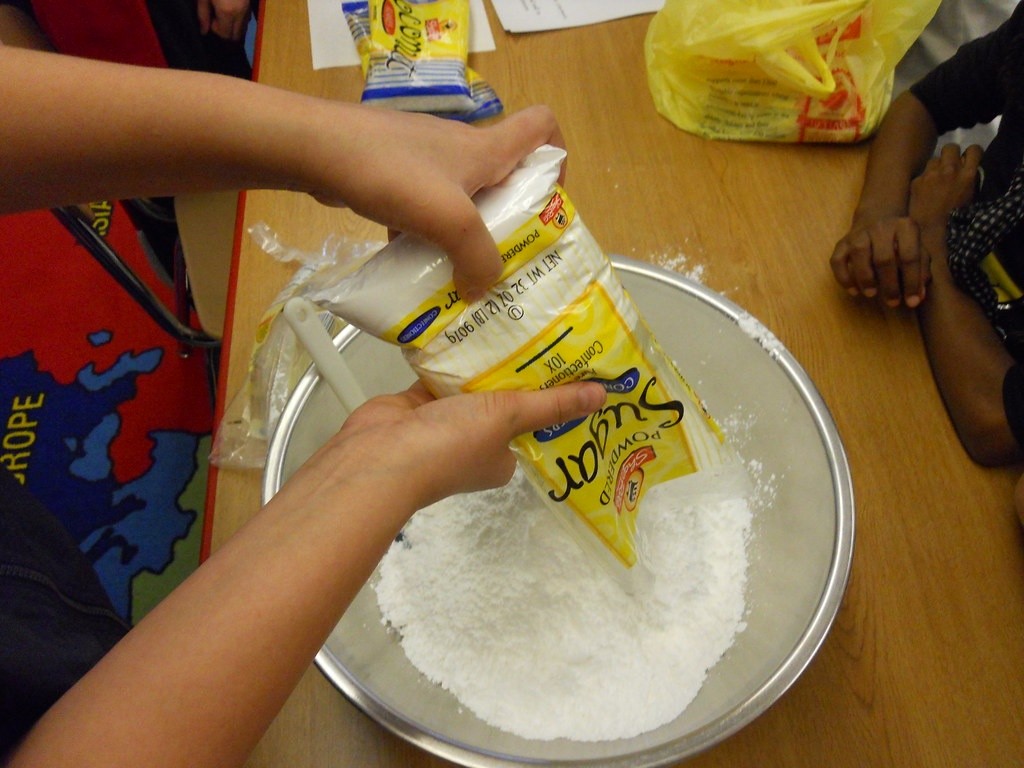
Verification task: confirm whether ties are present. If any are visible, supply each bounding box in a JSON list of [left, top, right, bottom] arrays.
[[948, 160, 1024, 318]]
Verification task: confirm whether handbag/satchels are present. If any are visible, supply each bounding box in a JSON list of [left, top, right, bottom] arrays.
[[645, 0, 942, 142]]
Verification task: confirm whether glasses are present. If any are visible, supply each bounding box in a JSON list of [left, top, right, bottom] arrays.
[[973, 152, 1015, 201]]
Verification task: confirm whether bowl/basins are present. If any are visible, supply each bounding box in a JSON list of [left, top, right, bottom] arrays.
[[261, 253, 855, 768]]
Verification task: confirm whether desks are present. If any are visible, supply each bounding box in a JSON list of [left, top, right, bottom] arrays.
[[199, 0, 1024, 768]]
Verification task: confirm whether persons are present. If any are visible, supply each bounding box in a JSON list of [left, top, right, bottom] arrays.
[[0, 1, 608, 768], [830, 1, 1024, 465]]
